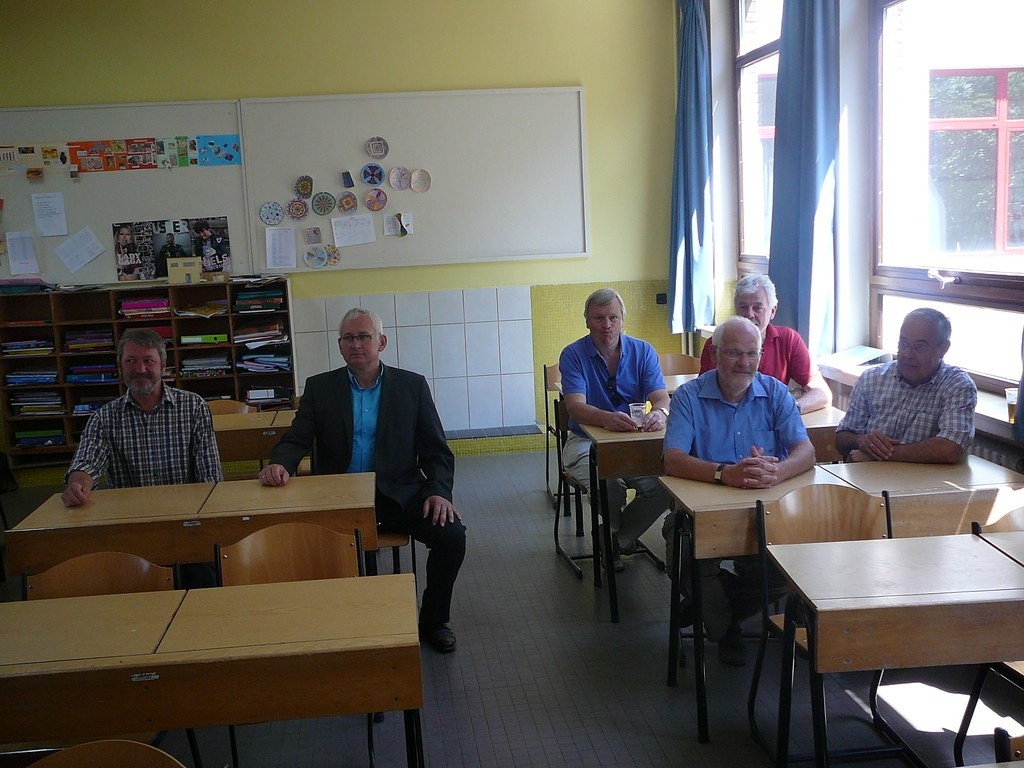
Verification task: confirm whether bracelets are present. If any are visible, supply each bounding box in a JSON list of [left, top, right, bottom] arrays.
[[795, 401, 802, 414], [714, 461, 737, 486], [659, 408, 669, 417]]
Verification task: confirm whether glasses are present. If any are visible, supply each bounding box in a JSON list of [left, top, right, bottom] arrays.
[[339, 332, 380, 345], [734, 302, 772, 315], [896, 339, 944, 355], [607, 375, 623, 407], [715, 345, 763, 360]]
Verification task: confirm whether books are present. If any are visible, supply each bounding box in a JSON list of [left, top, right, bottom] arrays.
[[229, 287, 293, 412], [62, 331, 120, 416], [118, 293, 175, 376], [0, 321, 65, 446], [173, 295, 232, 378]]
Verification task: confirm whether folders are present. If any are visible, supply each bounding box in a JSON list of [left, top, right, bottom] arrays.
[[180, 334, 228, 343], [235, 350, 292, 373], [66, 374, 113, 383]]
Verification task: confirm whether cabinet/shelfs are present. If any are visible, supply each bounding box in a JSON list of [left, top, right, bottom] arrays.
[[0, 275, 298, 470]]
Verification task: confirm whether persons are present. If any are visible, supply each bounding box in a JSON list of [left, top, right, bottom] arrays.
[[60, 330, 227, 589], [159, 233, 187, 261], [113, 225, 144, 281], [193, 220, 229, 272], [833, 308, 979, 464], [559, 289, 673, 571], [697, 274, 833, 415], [257, 307, 467, 651], [661, 315, 819, 674]]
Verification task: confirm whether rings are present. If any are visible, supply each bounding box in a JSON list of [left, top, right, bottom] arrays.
[[261, 475, 266, 479], [869, 443, 874, 447]]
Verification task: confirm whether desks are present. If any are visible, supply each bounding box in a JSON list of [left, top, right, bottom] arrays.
[[210, 410, 304, 483], [4, 470, 380, 575], [762, 529, 1022, 768], [1, 568, 426, 768], [554, 372, 705, 538], [655, 456, 1023, 743], [572, 401, 847, 624]]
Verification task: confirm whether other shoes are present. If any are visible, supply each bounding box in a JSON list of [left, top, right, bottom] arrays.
[[677, 597, 694, 628], [591, 525, 625, 572], [616, 529, 637, 555], [716, 603, 747, 666]]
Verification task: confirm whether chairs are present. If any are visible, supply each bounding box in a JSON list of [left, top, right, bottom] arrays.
[[20, 348, 1023, 767]]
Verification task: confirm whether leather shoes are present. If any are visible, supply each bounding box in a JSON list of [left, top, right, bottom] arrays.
[[431, 622, 457, 653]]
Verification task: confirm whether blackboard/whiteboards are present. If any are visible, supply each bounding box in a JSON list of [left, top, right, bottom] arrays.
[[0, 99, 254, 286], [241, 85, 592, 275]]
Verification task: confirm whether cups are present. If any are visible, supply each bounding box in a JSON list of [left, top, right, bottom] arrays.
[[629, 403, 647, 428]]
